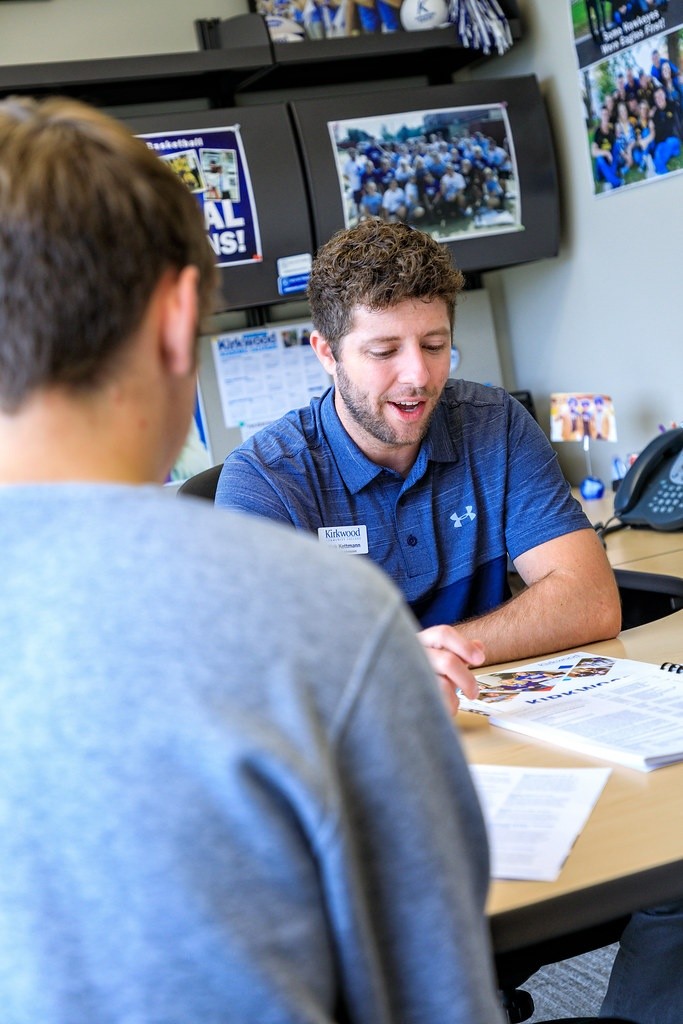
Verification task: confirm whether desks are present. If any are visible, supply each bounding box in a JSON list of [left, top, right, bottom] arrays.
[[440, 488, 682, 1024]]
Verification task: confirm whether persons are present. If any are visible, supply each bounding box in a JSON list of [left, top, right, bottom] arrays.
[[212, 216, 622, 718], [553, 396, 614, 441], [0, 95, 509, 1024], [253, 0, 683, 240]]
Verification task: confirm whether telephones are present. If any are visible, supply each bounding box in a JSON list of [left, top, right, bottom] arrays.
[[613, 427, 683, 531]]
